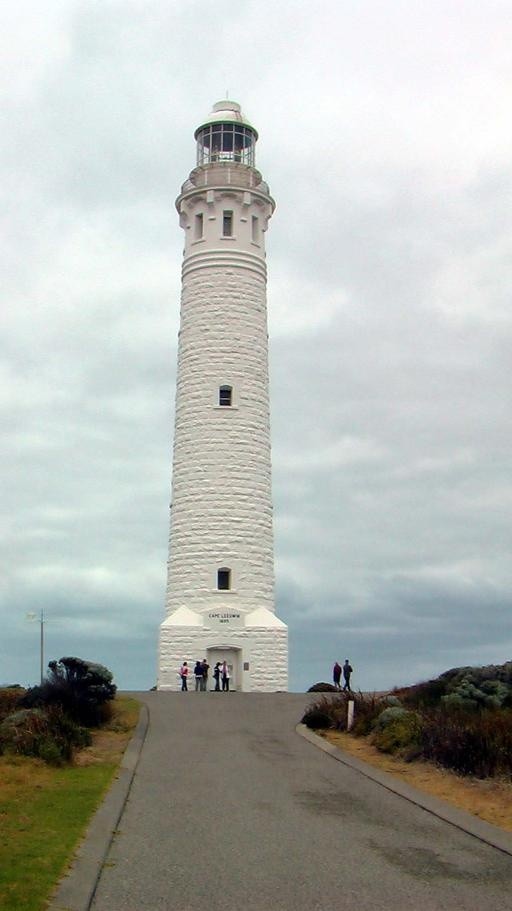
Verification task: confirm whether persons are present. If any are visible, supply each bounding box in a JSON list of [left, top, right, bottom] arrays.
[[333, 661, 342, 689], [343, 659, 353, 693], [213, 661, 222, 692], [194, 660, 204, 691], [180, 661, 189, 691], [220, 660, 231, 691], [201, 659, 209, 692]]
